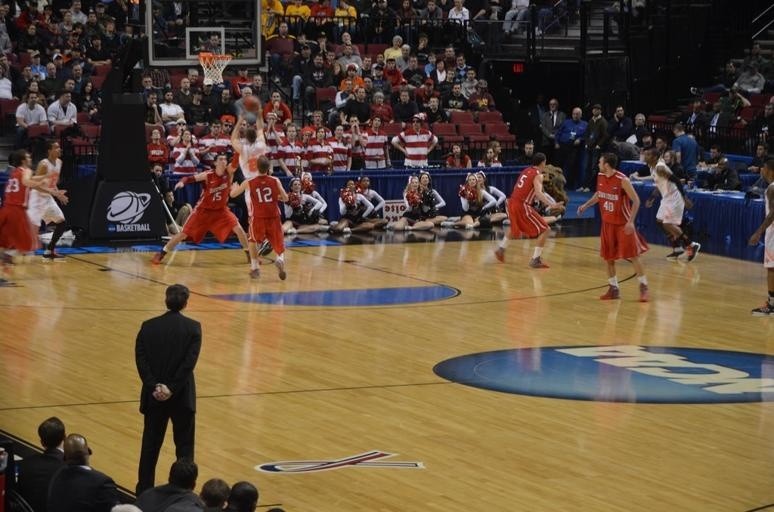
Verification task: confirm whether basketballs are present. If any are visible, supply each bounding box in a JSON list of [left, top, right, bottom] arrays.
[[244, 96, 260, 113]]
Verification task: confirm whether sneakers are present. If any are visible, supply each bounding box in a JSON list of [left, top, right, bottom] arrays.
[[666, 245, 686, 259], [639, 281, 651, 302], [493, 247, 505, 264], [275, 256, 287, 281], [250, 265, 261, 279], [153, 250, 166, 264], [600, 283, 620, 299], [750, 301, 774, 316], [686, 241, 701, 262], [42, 248, 66, 260], [529, 256, 551, 269], [247, 255, 264, 265]]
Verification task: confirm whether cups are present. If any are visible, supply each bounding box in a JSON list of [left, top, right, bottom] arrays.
[[687, 181, 694, 191], [680, 178, 685, 187]]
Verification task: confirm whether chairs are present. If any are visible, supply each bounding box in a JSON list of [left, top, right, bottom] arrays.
[[1, 2, 774, 258]]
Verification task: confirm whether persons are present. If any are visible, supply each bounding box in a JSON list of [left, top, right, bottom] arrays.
[[387, 172, 448, 230], [223, 480, 258, 512], [331, 176, 388, 232], [641, 148, 702, 263], [25, 140, 71, 260], [230, 155, 289, 281], [492, 152, 566, 270], [441, 171, 507, 229], [259, 0, 669, 45], [0, 1, 222, 145], [530, 94, 740, 190], [681, 43, 774, 170], [132, 279, 203, 498], [230, 106, 274, 257], [745, 160, 774, 317], [150, 149, 263, 266], [0, 148, 71, 289], [16, 417, 68, 511], [503, 188, 561, 224], [142, 22, 535, 189], [576, 151, 651, 303], [198, 478, 230, 511], [133, 456, 205, 511], [282, 173, 329, 233], [46, 433, 119, 511], [165, 189, 192, 234]]
